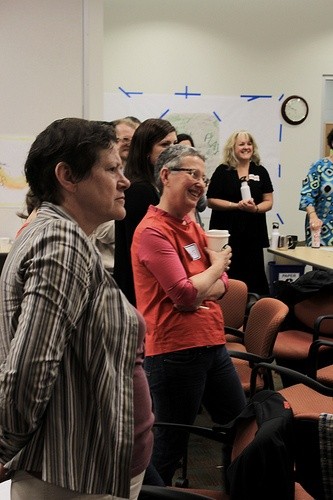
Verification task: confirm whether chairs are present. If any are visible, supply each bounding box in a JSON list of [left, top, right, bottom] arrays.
[[138, 390, 315, 500], [294, 285, 332, 338], [220, 279, 248, 341], [221, 297, 288, 391], [312, 315, 332, 386], [251, 340, 333, 422]]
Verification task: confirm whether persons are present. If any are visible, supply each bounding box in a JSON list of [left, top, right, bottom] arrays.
[[206, 131, 273, 332], [89, 118, 141, 278], [0, 117, 155, 500], [299, 128, 333, 247], [130, 145, 248, 500], [174, 134, 207, 230], [113, 119, 179, 307]]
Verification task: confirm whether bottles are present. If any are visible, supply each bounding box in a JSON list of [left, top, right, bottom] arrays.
[[311, 227, 321, 248], [271, 222, 280, 249], [240, 182, 251, 202]]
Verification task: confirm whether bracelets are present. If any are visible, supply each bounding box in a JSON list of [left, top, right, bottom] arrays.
[[308, 211, 317, 217], [229, 201, 234, 210], [255, 205, 259, 213]]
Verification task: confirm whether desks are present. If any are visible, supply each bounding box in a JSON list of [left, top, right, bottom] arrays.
[[268, 241, 333, 271]]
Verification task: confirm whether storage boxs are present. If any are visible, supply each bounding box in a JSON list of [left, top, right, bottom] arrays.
[[269, 261, 305, 282]]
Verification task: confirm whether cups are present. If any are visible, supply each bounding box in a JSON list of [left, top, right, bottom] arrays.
[[288, 235, 298, 249], [205, 229, 231, 252], [278, 235, 293, 250]]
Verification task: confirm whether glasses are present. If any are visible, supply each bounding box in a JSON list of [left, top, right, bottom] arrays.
[[116, 137, 133, 143], [168, 166, 210, 186]]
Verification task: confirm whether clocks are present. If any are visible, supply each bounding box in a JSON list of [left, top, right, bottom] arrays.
[[281, 94, 308, 125]]
